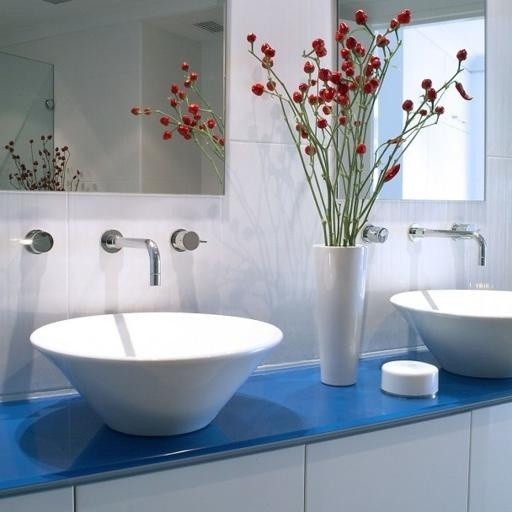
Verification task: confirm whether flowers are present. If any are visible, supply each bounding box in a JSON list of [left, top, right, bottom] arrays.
[[132, 62, 225, 189], [5, 135, 98, 192], [247, 0, 476, 247]]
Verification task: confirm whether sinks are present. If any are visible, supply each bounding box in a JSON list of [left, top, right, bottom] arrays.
[[388, 289, 511, 382], [29, 312, 285, 439]]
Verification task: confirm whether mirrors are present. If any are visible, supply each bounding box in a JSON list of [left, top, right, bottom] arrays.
[[337, 2, 486, 203], [2, 1, 229, 194]]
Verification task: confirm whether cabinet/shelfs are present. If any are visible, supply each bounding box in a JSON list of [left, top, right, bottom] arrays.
[[0, 346, 511, 512]]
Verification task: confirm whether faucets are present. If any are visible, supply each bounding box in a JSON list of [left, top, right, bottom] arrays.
[[100, 229, 162, 287], [409, 225, 487, 268]]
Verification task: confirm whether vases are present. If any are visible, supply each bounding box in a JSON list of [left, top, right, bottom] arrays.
[[313, 242, 370, 387]]
